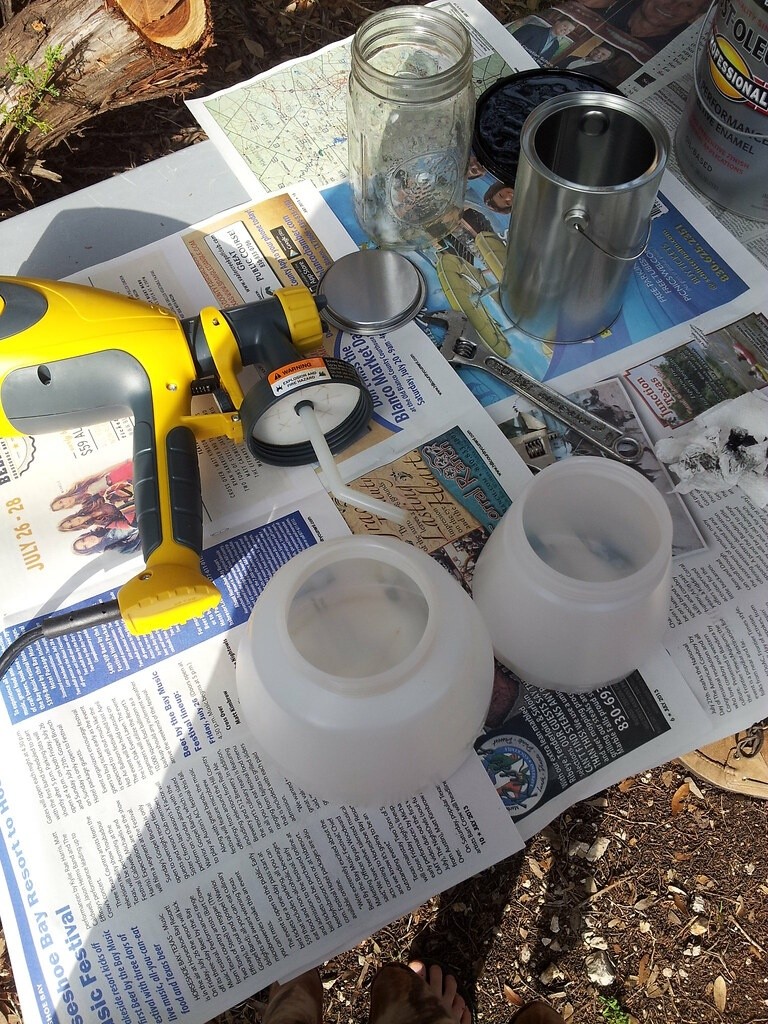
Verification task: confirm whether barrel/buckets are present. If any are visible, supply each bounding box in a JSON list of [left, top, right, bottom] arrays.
[[674, 0, 768, 225], [497, 92, 670, 346]]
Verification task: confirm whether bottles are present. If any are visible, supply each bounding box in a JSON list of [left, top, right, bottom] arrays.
[[233, 536, 494, 810], [346, 6, 476, 252], [469, 454, 673, 697]]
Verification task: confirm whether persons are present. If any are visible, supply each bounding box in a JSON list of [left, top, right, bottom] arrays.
[[512, 17, 577, 67], [483, 181, 514, 214], [466, 154, 486, 180], [612, 0, 709, 52], [555, 43, 615, 79], [51, 460, 142, 554]]
